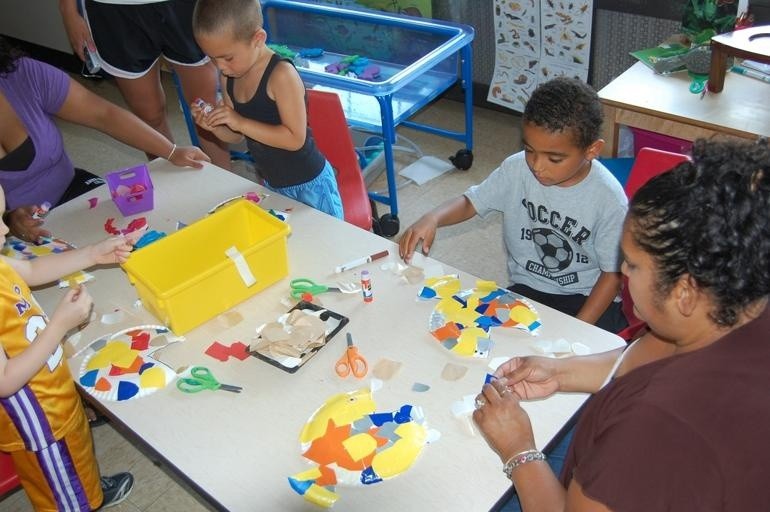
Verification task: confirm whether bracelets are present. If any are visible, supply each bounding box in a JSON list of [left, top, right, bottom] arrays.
[[167, 144, 176, 161], [503, 449, 546, 480]]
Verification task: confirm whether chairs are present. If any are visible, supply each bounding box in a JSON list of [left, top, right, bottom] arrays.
[[0, 450, 22, 495], [616, 148, 693, 339], [306, 89, 374, 234]]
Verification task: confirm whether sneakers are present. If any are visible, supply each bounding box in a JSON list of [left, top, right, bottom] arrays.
[[84, 403, 109, 427], [99, 472, 135, 509]]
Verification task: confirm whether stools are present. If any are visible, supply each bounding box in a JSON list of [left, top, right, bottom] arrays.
[[708, 25, 770, 93]]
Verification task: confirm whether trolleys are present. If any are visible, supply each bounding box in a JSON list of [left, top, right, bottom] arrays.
[[173, 3, 478, 236]]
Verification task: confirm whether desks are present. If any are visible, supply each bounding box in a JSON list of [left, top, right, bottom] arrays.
[[596, 41, 769, 157], [26, 157, 628, 512]]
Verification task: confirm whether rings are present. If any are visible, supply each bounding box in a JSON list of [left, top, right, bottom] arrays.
[[474, 399, 487, 409], [499, 385, 513, 395]]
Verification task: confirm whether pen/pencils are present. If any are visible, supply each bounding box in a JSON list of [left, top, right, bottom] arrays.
[[334, 249, 389, 274]]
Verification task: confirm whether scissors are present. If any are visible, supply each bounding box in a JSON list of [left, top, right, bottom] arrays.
[[176, 367, 242, 393], [290, 278, 342, 300], [334, 332, 368, 378]]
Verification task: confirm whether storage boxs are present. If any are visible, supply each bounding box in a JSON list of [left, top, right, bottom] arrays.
[[120, 200, 291, 335]]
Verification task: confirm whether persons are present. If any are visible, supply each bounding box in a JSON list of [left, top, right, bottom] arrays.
[[0, 187, 136, 512], [0, 39, 212, 213], [58, 0, 232, 172], [399, 76, 630, 336], [472, 134, 770, 512], [189, 0, 345, 222]]
